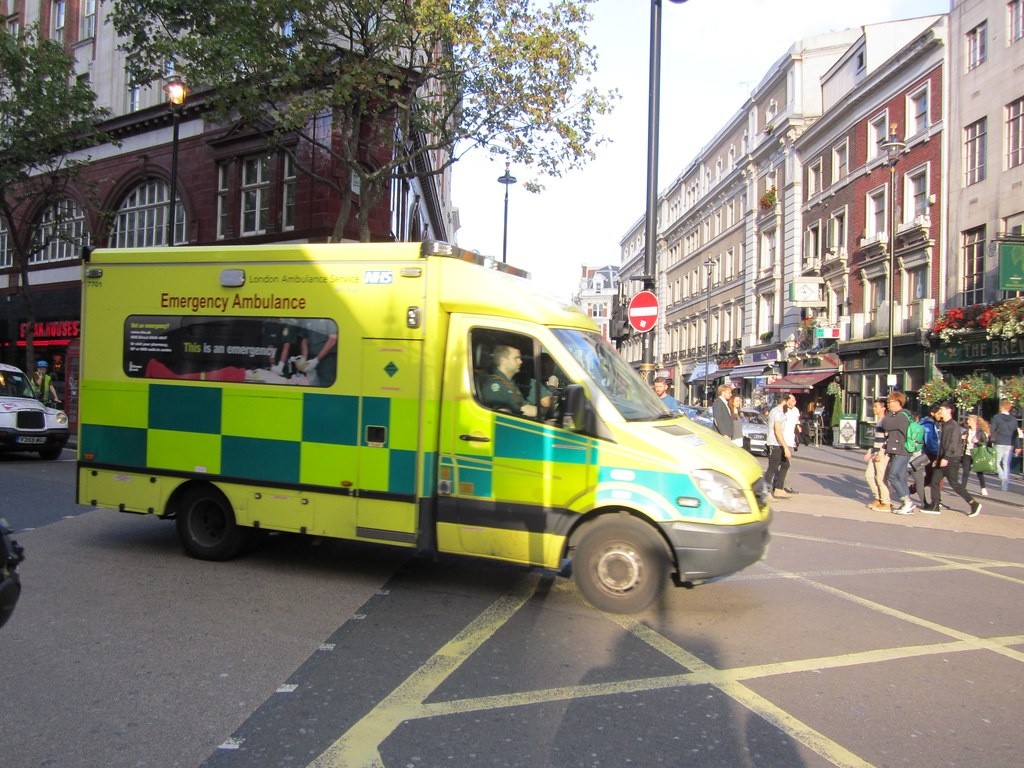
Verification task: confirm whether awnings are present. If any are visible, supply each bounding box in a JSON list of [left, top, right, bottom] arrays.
[[729, 366, 768, 378], [0, 285, 80, 348], [693, 370, 730, 384], [763, 373, 839, 393]]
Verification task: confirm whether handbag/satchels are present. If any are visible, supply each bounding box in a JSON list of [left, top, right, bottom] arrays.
[[969, 442, 998, 472], [910, 454, 931, 471]]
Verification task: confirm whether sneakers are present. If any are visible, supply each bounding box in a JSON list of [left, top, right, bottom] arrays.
[[920, 503, 940, 514], [868, 500, 881, 508], [893, 501, 916, 515], [968, 501, 982, 517], [872, 501, 890, 512]]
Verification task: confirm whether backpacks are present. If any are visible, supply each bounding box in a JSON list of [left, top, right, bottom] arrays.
[[898, 412, 925, 452]]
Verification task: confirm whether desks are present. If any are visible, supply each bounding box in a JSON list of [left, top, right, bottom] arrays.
[[810, 425, 830, 445]]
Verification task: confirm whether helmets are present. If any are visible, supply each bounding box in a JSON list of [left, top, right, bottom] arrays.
[[37, 361, 48, 367]]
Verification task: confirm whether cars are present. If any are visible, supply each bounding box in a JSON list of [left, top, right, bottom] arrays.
[[676, 399, 768, 459]]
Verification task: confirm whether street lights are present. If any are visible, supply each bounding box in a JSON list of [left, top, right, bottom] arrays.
[[496, 152, 517, 263], [162, 73, 193, 247], [878, 122, 906, 395]]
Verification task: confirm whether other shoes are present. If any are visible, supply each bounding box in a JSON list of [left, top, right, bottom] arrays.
[[767, 492, 777, 502], [938, 502, 950, 510], [784, 487, 799, 494], [982, 487, 988, 495], [773, 487, 790, 498]]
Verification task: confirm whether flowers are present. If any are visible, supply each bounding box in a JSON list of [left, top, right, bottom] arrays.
[[928, 296, 1024, 344], [914, 372, 1024, 410], [758, 185, 776, 209]]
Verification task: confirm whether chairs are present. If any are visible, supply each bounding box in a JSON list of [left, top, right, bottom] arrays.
[[474, 340, 490, 405], [801, 421, 828, 446]]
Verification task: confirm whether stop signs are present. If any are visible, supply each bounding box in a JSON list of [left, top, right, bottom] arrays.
[[627, 291, 660, 333]]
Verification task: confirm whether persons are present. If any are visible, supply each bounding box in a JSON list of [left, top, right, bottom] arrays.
[[728, 395, 743, 447], [765, 395, 802, 502], [712, 385, 734, 440], [865, 392, 1024, 515], [482, 343, 560, 424], [23, 361, 62, 404], [655, 377, 678, 410], [755, 397, 769, 419]]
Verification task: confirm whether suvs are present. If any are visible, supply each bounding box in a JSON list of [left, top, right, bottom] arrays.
[[0, 363, 71, 460]]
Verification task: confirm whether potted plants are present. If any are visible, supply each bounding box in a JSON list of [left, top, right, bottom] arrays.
[[760, 332, 773, 343]]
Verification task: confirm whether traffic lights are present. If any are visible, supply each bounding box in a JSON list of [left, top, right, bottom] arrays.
[[624, 324, 634, 339], [609, 319, 630, 342], [617, 305, 629, 322]]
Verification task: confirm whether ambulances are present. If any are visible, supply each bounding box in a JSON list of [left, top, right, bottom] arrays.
[[78, 239, 775, 615]]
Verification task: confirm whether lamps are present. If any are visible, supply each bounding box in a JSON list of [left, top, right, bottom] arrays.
[[877, 349, 887, 356], [787, 351, 824, 363]]
